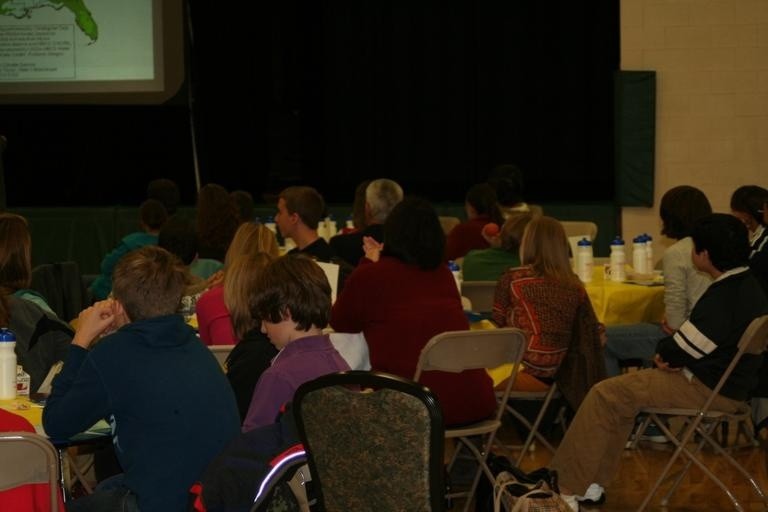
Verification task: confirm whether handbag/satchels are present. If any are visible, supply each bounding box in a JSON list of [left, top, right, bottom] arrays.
[[441, 454, 572, 512]]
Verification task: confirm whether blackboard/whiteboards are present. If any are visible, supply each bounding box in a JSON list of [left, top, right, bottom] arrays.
[[6, 71, 657, 207]]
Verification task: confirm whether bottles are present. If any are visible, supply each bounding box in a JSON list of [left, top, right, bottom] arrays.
[[632, 232, 653, 274], [15, 366, 30, 411], [610, 236, 625, 283], [447, 260, 461, 295], [576, 237, 594, 283], [252, 213, 354, 253], [0, 327, 18, 401]]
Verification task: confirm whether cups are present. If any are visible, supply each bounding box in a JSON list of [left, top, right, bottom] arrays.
[[30, 392, 48, 403]]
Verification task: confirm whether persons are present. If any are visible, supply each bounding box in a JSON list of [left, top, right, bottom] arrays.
[[0, 179, 768, 512]]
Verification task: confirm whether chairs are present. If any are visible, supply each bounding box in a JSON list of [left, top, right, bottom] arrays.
[[0, 431, 58, 511]]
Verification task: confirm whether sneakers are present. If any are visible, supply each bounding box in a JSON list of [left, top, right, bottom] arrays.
[[630, 419, 670, 442]]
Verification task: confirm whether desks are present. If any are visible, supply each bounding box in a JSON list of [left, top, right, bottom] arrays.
[[1, 393, 110, 494]]
